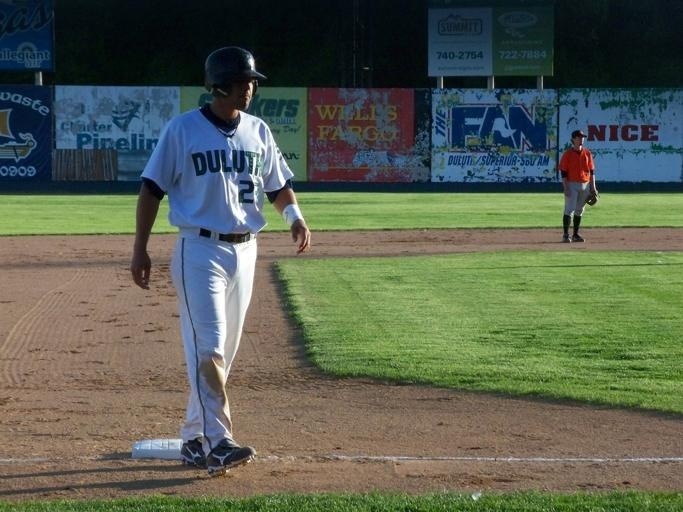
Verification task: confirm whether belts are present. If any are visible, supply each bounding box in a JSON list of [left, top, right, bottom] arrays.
[[199, 228, 251, 244]]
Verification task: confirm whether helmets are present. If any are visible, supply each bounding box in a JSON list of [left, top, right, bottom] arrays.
[[205, 46, 265, 91]]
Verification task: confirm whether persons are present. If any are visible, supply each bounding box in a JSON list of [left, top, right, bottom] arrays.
[[559, 130, 596, 243], [129, 45, 310, 476]]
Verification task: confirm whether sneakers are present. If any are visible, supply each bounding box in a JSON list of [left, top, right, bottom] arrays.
[[563, 234, 572, 242], [572, 235, 584, 242], [180, 438, 207, 469], [206, 443, 256, 477]]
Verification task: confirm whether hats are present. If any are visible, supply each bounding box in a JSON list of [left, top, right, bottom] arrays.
[[572, 130, 587, 138]]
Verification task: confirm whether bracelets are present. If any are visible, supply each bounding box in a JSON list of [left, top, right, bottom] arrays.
[[282, 204, 305, 228]]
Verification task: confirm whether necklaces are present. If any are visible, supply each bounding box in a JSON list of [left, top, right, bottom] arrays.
[[208, 110, 238, 137]]
